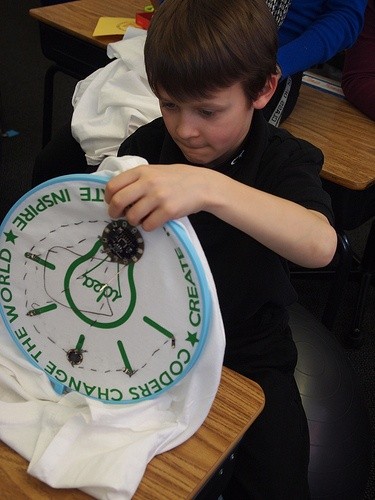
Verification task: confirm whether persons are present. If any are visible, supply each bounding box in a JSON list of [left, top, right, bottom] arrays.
[[104, 0, 340, 500], [261, 0, 369, 128]]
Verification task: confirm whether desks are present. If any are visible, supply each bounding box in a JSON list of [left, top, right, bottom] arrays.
[[27, 0, 375, 349], [0, 366, 265, 499]]
